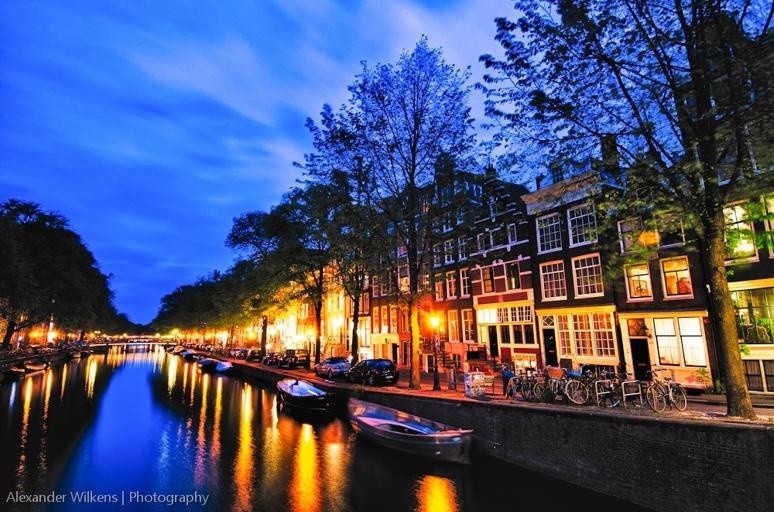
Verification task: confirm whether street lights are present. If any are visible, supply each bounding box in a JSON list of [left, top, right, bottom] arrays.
[[426, 315, 440, 388]]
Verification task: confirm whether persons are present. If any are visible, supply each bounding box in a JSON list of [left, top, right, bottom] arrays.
[[502, 365, 514, 396], [588, 370, 594, 378], [519, 370, 523, 375]]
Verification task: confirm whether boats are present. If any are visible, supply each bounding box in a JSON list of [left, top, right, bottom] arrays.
[[278, 379, 336, 414], [347, 398, 474, 465], [166, 345, 183, 354], [217, 360, 232, 371], [79, 347, 94, 356], [9, 361, 51, 375]]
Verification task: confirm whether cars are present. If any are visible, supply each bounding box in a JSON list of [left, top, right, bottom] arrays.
[[315, 356, 351, 378], [182, 343, 283, 366], [349, 360, 400, 386]]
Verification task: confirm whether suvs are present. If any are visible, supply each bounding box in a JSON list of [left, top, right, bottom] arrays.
[[278, 348, 310, 369]]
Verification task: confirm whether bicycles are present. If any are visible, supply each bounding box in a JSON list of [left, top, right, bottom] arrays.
[[507, 367, 645, 409], [646, 370, 688, 414]]
